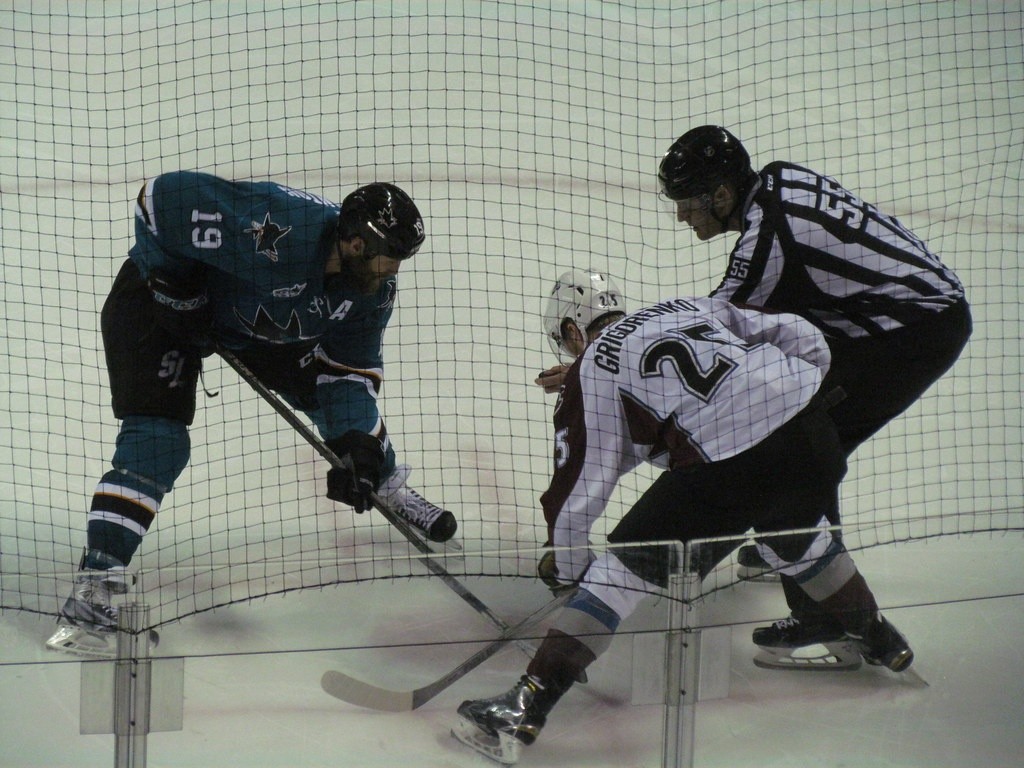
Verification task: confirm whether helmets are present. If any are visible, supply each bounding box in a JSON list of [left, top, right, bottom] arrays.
[[543, 271, 628, 348], [658, 126, 754, 200], [338, 182, 426, 260]]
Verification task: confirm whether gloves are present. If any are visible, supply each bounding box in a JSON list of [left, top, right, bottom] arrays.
[[538, 551, 591, 598], [319, 429, 387, 513], [146, 267, 216, 358]]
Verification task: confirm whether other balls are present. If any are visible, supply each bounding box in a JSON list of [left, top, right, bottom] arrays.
[[538, 370, 556, 379]]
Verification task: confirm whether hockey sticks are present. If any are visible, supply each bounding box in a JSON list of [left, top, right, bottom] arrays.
[[316, 586, 579, 714], [212, 341, 634, 708]]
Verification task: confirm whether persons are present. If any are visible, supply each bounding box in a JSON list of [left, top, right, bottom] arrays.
[[535, 126, 975, 669], [450, 268, 929, 764], [48, 171, 464, 659]]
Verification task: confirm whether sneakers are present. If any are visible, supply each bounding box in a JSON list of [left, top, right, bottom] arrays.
[[371, 464, 467, 559], [843, 608, 931, 687], [45, 546, 160, 658], [450, 674, 562, 764], [751, 610, 863, 670], [735, 539, 782, 583]]
